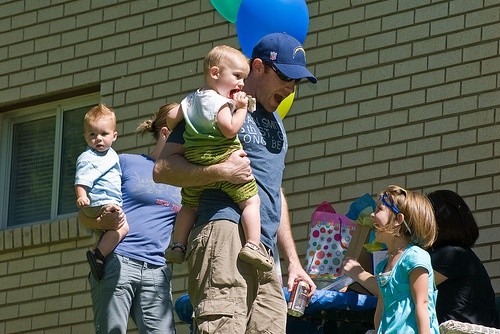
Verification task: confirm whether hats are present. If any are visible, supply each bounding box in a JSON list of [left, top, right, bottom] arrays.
[[251, 32, 319, 84]]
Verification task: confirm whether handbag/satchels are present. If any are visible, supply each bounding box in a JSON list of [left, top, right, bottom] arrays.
[[304, 210, 362, 291]]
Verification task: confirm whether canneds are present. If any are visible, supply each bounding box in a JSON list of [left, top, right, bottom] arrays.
[[287, 279, 311, 317]]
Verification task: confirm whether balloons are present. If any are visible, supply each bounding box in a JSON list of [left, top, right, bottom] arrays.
[[210, 0, 240, 24], [237, 0, 309, 59]]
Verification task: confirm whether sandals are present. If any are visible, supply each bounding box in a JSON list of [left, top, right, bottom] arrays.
[[86, 248, 106, 281]]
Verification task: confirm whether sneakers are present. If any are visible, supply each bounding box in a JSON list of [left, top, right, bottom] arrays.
[[164, 244, 184, 264], [239, 242, 275, 271]]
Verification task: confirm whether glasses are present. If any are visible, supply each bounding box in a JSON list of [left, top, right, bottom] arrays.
[[265, 62, 303, 84]]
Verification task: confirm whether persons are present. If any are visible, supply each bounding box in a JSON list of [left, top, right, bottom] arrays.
[[153, 33, 317, 334], [370, 184, 440, 334], [78, 102, 182, 334], [166, 45, 273, 272], [343, 190, 495, 327], [74, 104, 129, 280]]
